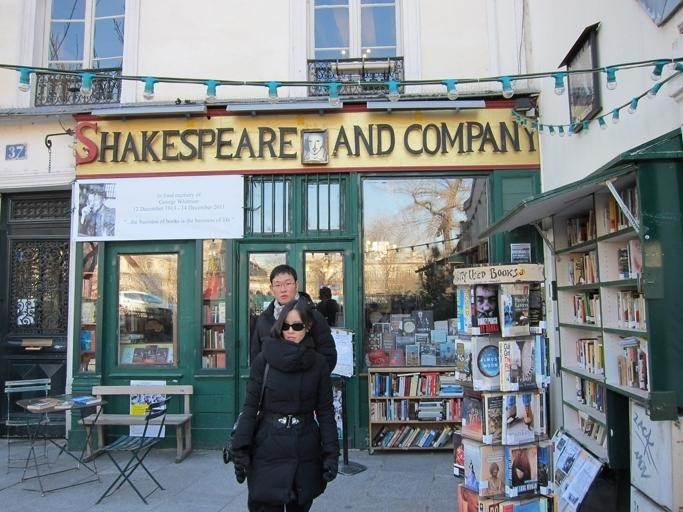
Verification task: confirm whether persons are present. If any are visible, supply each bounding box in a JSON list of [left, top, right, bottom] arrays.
[[368, 301, 383, 328], [249, 267, 337, 376], [232, 300, 339, 512], [78, 183, 115, 239], [304, 131, 326, 163]]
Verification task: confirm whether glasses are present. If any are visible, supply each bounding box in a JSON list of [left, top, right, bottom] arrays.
[[282, 323, 305, 331], [272, 282, 296, 287]]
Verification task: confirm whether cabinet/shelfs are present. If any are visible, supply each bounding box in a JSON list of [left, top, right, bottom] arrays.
[[80, 271, 226, 369], [368, 173, 683, 512]]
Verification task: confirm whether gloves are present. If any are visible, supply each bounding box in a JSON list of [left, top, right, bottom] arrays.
[[323, 452, 338, 481], [234, 453, 250, 483]]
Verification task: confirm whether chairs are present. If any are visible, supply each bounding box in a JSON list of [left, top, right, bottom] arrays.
[[94, 397, 172, 505], [5, 378, 51, 475]]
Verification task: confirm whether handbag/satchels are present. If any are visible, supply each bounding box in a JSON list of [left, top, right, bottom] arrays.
[[224, 412, 243, 463]]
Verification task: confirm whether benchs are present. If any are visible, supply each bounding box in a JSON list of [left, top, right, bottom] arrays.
[[78, 384, 194, 463]]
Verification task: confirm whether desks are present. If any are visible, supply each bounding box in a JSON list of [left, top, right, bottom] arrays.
[[16, 393, 107, 497]]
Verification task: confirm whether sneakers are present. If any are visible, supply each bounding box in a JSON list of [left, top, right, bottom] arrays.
[[507, 405, 516, 419], [524, 405, 533, 424]]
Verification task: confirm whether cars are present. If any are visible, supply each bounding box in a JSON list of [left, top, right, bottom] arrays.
[[119, 290, 173, 315]]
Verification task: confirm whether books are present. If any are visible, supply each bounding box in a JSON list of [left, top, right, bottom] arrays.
[[455, 188, 647, 511], [81, 277, 98, 371], [20, 395, 102, 412], [365, 311, 458, 448], [202, 303, 227, 369]]
